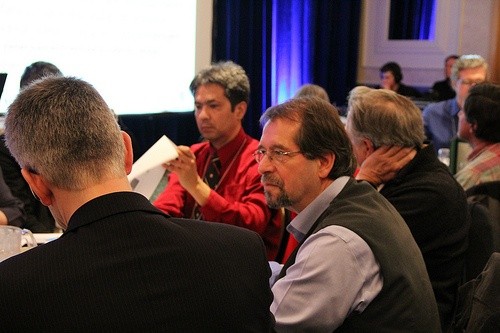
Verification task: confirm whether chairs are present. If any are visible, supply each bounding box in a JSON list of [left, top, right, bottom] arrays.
[[445, 194, 500, 333], [117, 111, 203, 162]]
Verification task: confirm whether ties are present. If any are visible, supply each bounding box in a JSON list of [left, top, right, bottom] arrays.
[[193, 152, 222, 222]]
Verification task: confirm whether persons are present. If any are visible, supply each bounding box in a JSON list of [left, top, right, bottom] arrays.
[[252, 96, 441, 333], [429, 55, 459, 103], [1, 74, 276, 332], [151, 61, 299, 265], [345, 90, 469, 333], [422, 54, 492, 175], [0, 62, 65, 234], [452, 81, 500, 252], [373, 62, 422, 100]]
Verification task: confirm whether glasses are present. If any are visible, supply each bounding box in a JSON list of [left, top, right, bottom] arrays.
[[253, 149, 304, 164]]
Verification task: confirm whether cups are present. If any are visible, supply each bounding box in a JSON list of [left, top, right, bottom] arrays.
[[0, 225, 38, 263]]
